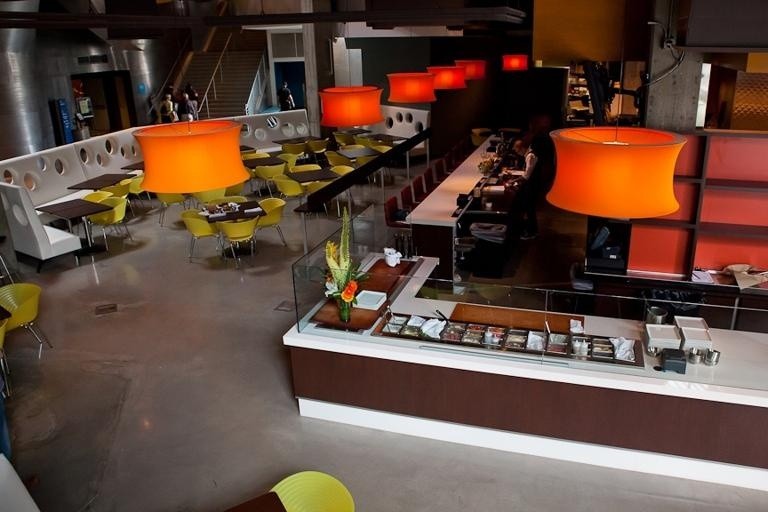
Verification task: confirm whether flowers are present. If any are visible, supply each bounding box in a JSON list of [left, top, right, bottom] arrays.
[[321, 205, 372, 307]]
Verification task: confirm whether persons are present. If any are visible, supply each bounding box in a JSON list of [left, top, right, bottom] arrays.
[[502, 139, 550, 240], [184, 81, 198, 111], [178, 93, 195, 121], [278, 82, 295, 111], [171, 111, 177, 122], [158, 95, 173, 122], [163, 84, 177, 111]]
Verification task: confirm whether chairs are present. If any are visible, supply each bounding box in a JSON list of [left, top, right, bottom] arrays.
[[191, 187, 227, 207], [324, 151, 353, 166], [443, 152, 460, 173], [156, 193, 187, 228], [272, 174, 307, 206], [86, 196, 134, 253], [332, 131, 350, 147], [282, 141, 309, 153], [99, 179, 138, 225], [225, 182, 246, 196], [399, 183, 421, 213], [255, 162, 286, 198], [1, 315, 13, 400], [423, 167, 441, 194], [254, 197, 287, 250], [459, 138, 477, 156], [451, 145, 467, 165], [383, 195, 413, 256], [306, 138, 330, 163], [307, 181, 330, 195], [353, 136, 393, 154], [76, 191, 114, 242], [330, 166, 356, 176], [241, 152, 271, 161], [207, 196, 248, 207], [289, 164, 323, 174], [0, 283, 42, 344], [412, 174, 431, 202], [216, 215, 260, 267], [276, 152, 305, 173], [119, 174, 154, 212], [434, 158, 451, 181], [180, 209, 220, 262]]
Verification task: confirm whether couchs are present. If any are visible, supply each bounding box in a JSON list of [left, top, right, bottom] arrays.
[[337, 105, 431, 181], [0, 107, 312, 273]]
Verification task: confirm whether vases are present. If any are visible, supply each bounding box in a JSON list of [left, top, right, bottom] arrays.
[[338, 299, 351, 324]]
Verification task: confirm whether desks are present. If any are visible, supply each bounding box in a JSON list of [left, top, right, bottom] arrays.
[[333, 145, 384, 169], [201, 200, 267, 259], [67, 174, 138, 192], [359, 133, 409, 142], [271, 135, 325, 145], [284, 168, 342, 184], [34, 198, 114, 266], [120, 161, 145, 171]]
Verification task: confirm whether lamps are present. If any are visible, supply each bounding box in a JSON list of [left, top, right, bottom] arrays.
[[544, 1, 688, 220], [130, 1, 252, 195], [387, 1, 437, 104], [502, 0, 528, 71], [428, 0, 466, 91], [318, 1, 383, 129], [456, 0, 486, 78]]
